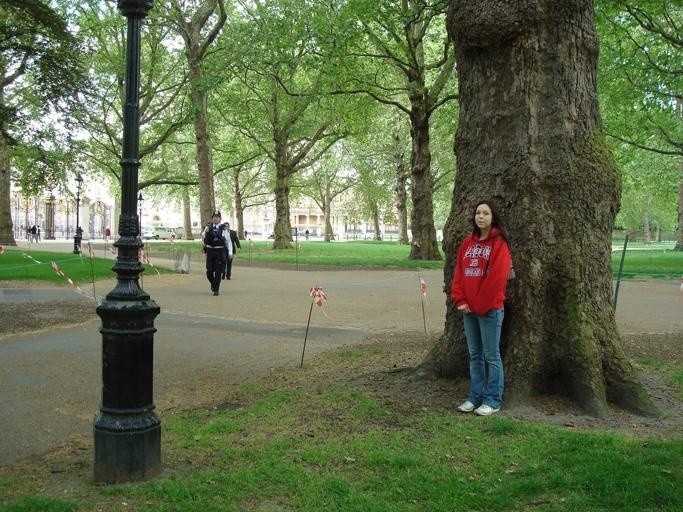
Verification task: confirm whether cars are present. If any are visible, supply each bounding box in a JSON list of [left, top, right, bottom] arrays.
[[361, 232, 376, 241]]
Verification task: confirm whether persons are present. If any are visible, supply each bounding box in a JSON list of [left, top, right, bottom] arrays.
[[451, 199, 510, 416], [201, 210, 233, 296], [221, 222, 240, 280], [305, 229, 309, 239], [17, 225, 111, 244], [244, 230, 247, 237]]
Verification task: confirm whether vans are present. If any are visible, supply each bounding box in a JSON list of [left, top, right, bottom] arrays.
[[143, 226, 177, 241]]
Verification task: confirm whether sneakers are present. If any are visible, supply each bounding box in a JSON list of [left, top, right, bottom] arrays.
[[455, 401, 476, 412], [473, 404, 500, 416]]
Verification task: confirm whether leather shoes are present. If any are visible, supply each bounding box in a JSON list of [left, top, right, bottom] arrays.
[[213, 292, 219, 296], [210, 284, 216, 292], [222, 275, 225, 280], [226, 276, 231, 280]]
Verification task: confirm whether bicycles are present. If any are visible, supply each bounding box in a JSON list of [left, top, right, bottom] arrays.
[[305, 233, 310, 241]]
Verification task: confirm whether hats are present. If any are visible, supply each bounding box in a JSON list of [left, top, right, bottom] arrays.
[[212, 210, 221, 219]]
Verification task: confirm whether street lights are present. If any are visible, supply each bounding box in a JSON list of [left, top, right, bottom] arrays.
[[87, 0, 164, 486], [137, 191, 145, 238], [71, 170, 85, 256]]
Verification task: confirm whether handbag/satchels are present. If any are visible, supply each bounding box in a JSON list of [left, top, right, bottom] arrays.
[[487, 237, 516, 280]]
